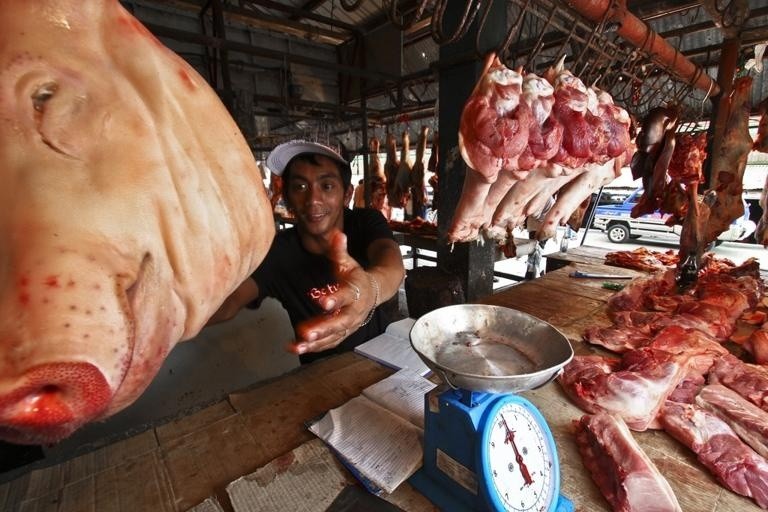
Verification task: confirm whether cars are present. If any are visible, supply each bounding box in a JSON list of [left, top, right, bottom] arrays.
[[418, 182, 436, 224]]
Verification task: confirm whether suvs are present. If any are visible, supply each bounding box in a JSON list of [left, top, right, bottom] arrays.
[[592, 185, 759, 254]]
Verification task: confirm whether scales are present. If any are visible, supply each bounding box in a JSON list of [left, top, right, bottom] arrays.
[[407, 301, 577, 512]]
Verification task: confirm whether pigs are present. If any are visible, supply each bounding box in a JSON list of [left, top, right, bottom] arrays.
[[1, 0, 278, 451]]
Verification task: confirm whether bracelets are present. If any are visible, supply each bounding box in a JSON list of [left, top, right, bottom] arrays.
[[362, 273, 380, 327]]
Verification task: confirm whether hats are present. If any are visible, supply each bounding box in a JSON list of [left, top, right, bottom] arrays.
[[265, 130, 351, 177]]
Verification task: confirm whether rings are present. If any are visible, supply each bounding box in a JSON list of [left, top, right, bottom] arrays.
[[348, 283, 360, 302], [344, 329, 349, 336]]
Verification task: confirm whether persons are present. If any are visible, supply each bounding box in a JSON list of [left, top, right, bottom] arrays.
[[202, 140, 405, 368], [350, 177, 427, 258]]
